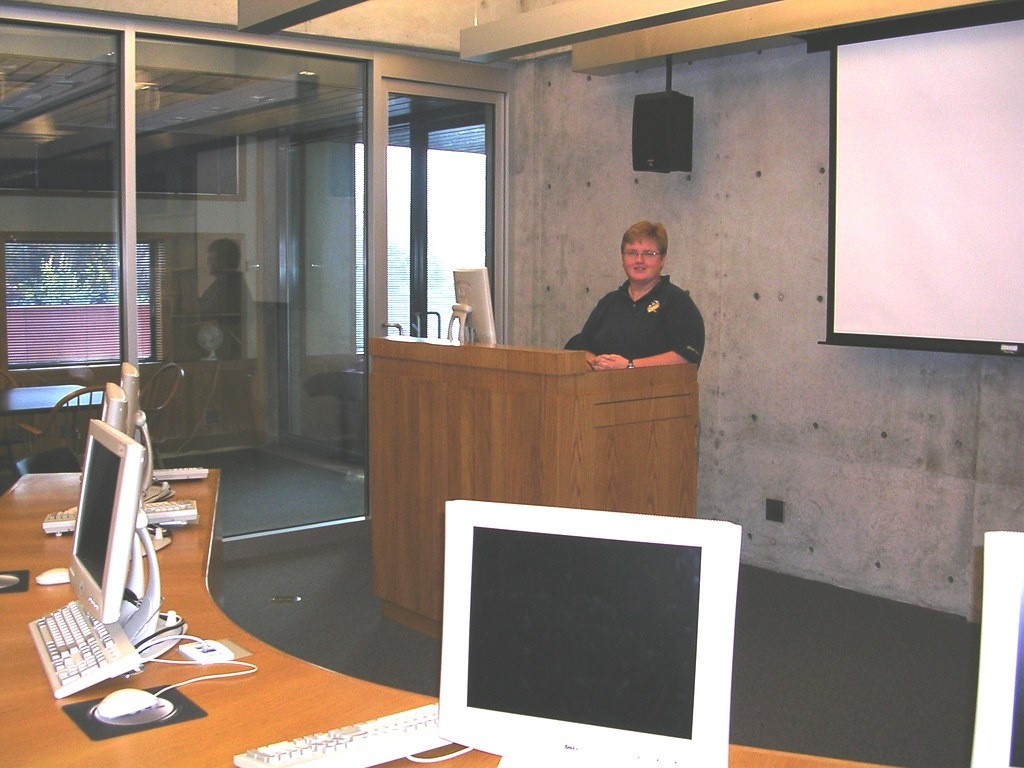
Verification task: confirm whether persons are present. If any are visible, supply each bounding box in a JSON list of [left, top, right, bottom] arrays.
[[563, 221, 705, 369], [183, 238, 246, 359]]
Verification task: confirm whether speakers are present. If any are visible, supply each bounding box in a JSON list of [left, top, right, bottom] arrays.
[[633, 90, 693, 173]]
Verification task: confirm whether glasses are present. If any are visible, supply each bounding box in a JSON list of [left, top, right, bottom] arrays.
[[622, 250, 663, 258]]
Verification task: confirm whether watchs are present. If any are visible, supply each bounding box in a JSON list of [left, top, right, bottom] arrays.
[[628, 358, 634, 368]]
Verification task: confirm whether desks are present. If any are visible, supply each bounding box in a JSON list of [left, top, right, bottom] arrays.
[[0, 467, 895, 768], [0, 384, 104, 456]]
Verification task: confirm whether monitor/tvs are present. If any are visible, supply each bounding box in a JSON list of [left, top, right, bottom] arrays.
[[971, 531, 1024, 768], [438, 500, 742, 768], [69, 363, 185, 663], [452, 268, 496, 345]]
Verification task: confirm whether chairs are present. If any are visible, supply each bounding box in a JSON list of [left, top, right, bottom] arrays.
[[0, 361, 191, 492]]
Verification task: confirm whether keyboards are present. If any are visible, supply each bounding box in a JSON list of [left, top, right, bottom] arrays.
[[43, 497, 198, 537], [153, 468, 209, 482], [234, 705, 451, 768], [28, 599, 143, 699]]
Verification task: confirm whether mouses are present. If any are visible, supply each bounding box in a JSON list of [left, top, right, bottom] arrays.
[[97, 688, 158, 719], [36, 567, 71, 586]]
